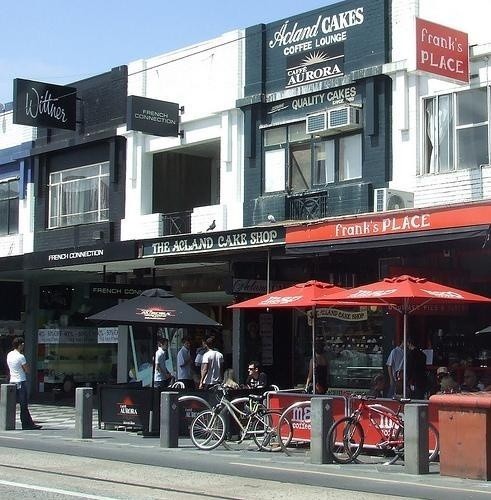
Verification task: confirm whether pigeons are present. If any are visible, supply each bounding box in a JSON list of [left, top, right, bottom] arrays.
[[206, 220, 216, 231], [266, 212, 276, 223]]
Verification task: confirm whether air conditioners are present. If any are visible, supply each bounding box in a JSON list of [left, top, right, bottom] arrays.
[[305, 103, 363, 136], [373, 188, 415, 215]]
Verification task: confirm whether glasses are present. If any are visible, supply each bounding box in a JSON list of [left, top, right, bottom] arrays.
[[247, 367, 256, 370]]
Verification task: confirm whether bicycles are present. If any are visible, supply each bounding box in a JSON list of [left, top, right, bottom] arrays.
[[325, 394, 439, 465], [190, 381, 293, 452]]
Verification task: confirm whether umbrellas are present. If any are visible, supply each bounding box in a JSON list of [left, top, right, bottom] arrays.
[[225, 279, 397, 395], [84, 287, 223, 433], [311, 275, 491, 400]]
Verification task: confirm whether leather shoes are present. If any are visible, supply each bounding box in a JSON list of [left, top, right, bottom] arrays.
[[22, 425, 40, 430]]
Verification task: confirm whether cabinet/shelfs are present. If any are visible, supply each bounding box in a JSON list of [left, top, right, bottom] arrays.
[[45, 343, 113, 392], [320, 315, 391, 387]]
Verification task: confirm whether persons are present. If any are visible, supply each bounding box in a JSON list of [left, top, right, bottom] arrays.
[[304, 335, 485, 401], [241, 359, 267, 390], [153, 335, 223, 389], [6, 337, 42, 430]]
